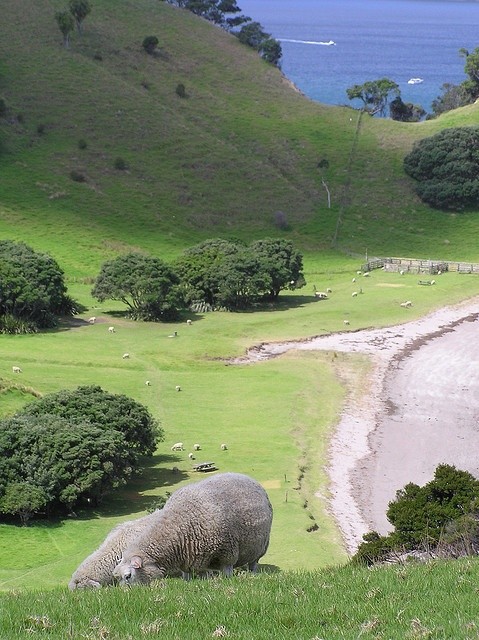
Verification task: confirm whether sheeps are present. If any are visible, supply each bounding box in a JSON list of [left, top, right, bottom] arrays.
[[112, 471, 274, 586], [69, 505, 169, 589]]
